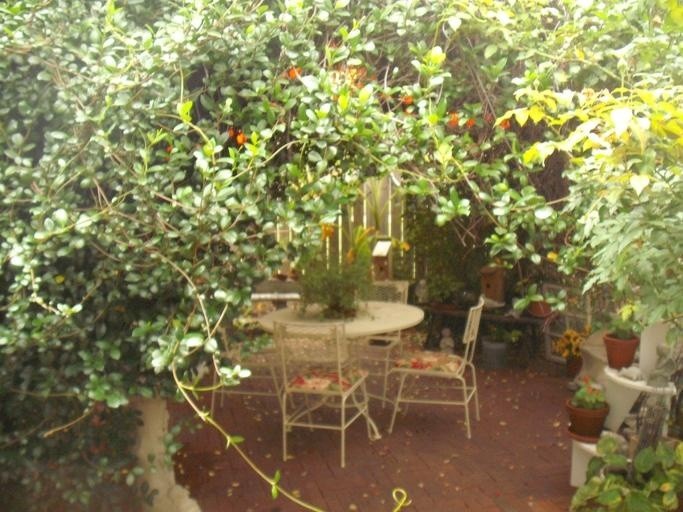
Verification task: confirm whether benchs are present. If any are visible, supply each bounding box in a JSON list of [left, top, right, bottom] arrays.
[[416, 294, 559, 364]]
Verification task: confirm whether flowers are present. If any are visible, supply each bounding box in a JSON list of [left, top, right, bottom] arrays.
[[551, 326, 589, 369], [571, 374, 606, 408]]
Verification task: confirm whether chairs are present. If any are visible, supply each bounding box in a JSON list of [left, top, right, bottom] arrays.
[[270, 317, 378, 468], [383, 292, 490, 441]]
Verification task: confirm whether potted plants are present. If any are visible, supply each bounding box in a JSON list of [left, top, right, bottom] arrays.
[[601, 306, 640, 370]]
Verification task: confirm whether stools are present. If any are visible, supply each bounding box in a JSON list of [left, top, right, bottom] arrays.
[[206, 347, 299, 428]]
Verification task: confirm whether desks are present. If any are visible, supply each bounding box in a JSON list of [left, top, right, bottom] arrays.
[[256, 297, 428, 425]]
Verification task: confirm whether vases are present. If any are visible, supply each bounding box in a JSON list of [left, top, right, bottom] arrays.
[[566, 357, 583, 374], [564, 397, 613, 446]]
